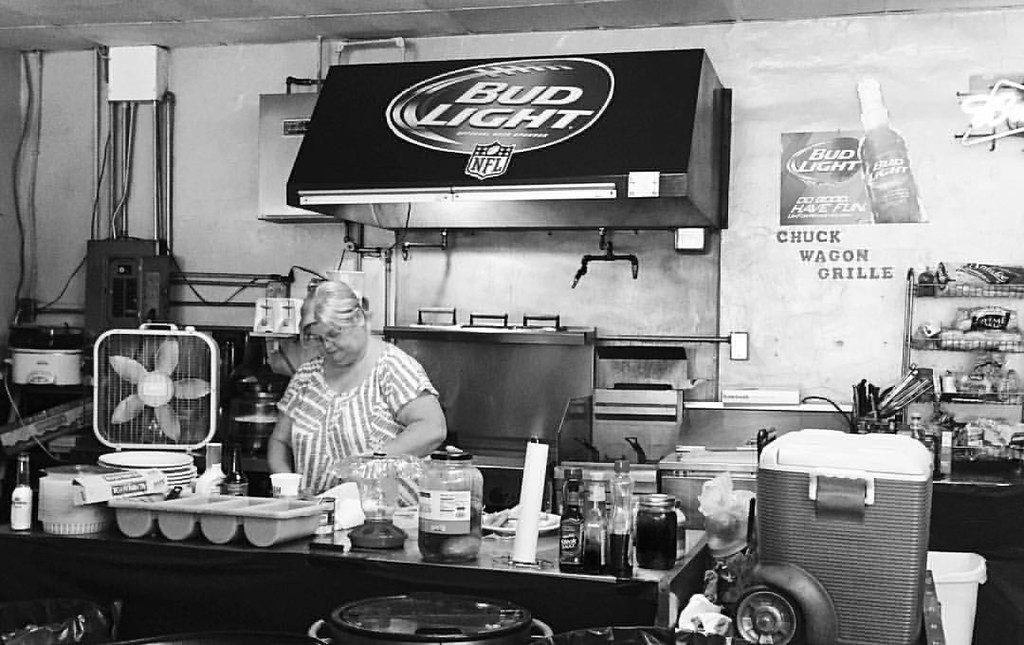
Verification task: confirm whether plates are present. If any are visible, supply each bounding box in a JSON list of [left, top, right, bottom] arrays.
[[97, 451, 197, 485], [480, 514, 561, 535]]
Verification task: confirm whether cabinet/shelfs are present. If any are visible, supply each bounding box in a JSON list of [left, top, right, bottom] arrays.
[[902, 267, 1024, 460]]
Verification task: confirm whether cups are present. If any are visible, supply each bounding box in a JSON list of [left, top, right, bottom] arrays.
[[270, 473, 302, 498]]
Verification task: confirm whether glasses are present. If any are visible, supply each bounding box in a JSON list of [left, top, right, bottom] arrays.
[[304, 326, 341, 348]]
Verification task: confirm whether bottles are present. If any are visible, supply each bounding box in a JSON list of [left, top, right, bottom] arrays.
[[314, 498, 335, 534], [557, 460, 685, 578], [10, 451, 34, 531], [858, 108, 919, 223], [418, 453, 485, 562], [909, 413, 925, 444], [220, 444, 248, 497]]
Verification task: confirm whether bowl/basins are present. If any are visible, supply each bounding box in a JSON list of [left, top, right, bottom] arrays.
[[39, 465, 113, 533]]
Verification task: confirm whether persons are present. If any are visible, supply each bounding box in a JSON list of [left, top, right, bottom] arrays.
[[266, 279, 447, 501]]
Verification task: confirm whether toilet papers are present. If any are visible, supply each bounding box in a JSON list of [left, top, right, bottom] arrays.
[[511, 441, 550, 564]]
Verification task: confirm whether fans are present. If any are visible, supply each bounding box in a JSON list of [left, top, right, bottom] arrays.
[[93, 324, 221, 453]]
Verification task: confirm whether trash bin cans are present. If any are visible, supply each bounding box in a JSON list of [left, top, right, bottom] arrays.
[[531, 627, 724, 645], [0, 596, 126, 645]]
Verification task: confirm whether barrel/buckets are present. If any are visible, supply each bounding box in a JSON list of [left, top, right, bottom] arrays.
[[757, 428, 934, 641], [923, 548, 988, 644]]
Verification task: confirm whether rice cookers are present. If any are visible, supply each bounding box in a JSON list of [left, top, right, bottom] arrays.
[[4, 325, 85, 386]]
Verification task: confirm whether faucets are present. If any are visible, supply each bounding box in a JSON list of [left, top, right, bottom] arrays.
[[571, 266, 585, 289], [631, 264, 639, 279]]
